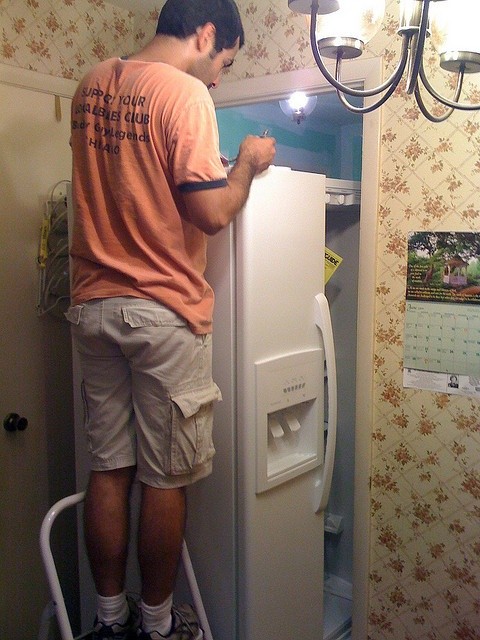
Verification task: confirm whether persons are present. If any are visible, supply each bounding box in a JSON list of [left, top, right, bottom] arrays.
[[62, 1, 275, 639]]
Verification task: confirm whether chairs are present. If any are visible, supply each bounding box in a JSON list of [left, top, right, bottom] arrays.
[[40, 488, 213, 640]]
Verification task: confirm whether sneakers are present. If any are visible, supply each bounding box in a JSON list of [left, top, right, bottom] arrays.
[[92, 591, 143, 640], [136, 602, 200, 639]]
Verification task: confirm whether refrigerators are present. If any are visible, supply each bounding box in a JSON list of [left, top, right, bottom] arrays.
[[67, 166, 358, 639]]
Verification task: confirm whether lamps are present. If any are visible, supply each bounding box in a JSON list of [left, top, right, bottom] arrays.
[[288, 0, 480, 121], [279, 95, 317, 124]]
